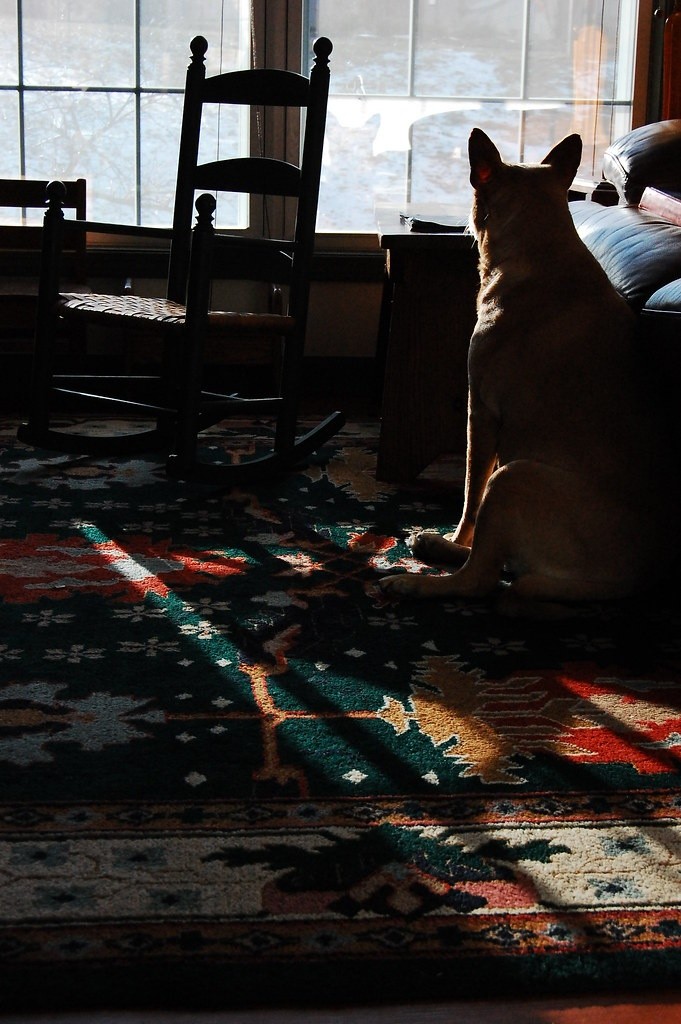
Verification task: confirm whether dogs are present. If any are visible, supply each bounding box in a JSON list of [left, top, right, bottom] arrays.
[[375, 127, 669, 606]]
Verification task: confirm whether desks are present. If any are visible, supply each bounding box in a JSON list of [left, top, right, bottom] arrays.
[[373, 202, 479, 482]]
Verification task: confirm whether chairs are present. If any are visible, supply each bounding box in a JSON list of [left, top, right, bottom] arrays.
[[0, 179, 96, 404], [16, 40, 347, 493]]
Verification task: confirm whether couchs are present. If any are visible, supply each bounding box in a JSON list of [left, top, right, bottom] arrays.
[[567, 117, 681, 388]]
